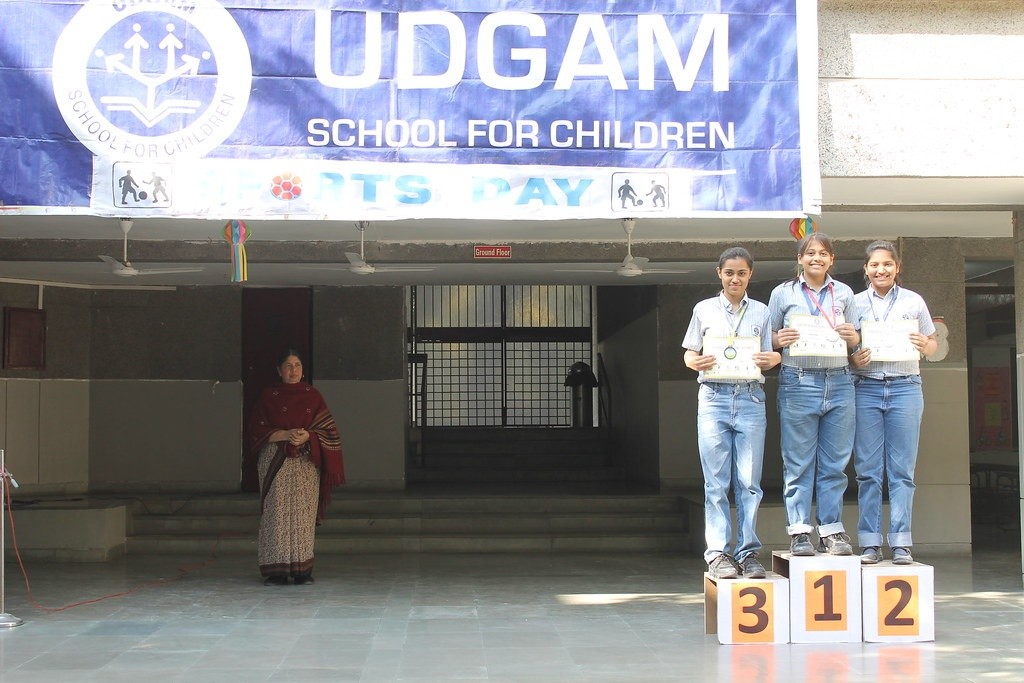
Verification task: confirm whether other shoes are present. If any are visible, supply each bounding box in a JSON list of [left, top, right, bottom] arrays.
[[294, 576, 314, 585], [264, 575, 289, 586]]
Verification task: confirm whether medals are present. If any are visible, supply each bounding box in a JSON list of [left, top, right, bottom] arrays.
[[725, 346, 736, 359], [827, 328, 838, 341]]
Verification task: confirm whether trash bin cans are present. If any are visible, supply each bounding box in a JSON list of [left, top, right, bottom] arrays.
[[563, 362, 599, 430]]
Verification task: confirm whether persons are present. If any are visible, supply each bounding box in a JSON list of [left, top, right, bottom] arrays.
[[849, 240, 937, 563], [768, 232, 860, 557], [681, 247, 782, 580], [245, 349, 345, 586]]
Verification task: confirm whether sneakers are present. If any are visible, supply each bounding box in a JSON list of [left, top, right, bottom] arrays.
[[892, 546, 914, 564], [737, 553, 767, 578], [861, 546, 884, 564], [708, 554, 739, 578], [817, 531, 854, 555], [790, 533, 816, 556]]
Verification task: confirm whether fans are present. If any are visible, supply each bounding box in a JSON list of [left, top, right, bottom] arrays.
[[555, 217, 697, 277], [93, 218, 203, 278], [293, 219, 439, 279]]
